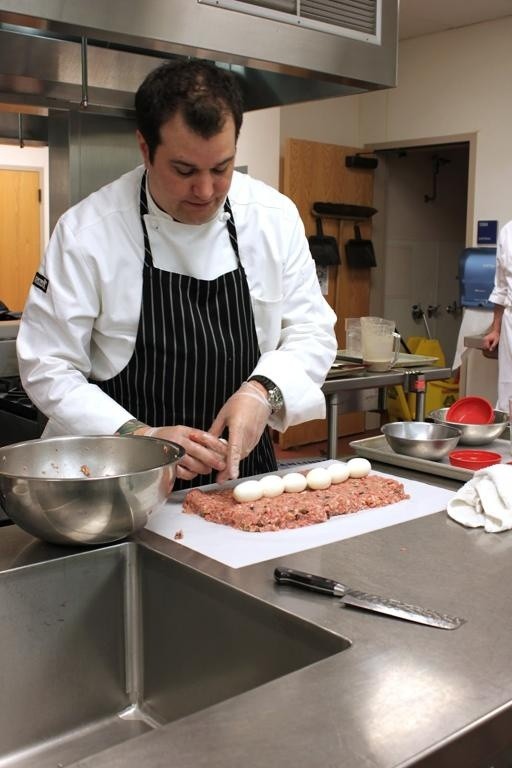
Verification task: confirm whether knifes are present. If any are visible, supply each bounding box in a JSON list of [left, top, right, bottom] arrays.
[[273, 565, 468, 631]]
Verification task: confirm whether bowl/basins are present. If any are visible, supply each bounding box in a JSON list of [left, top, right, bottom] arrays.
[[444, 396, 496, 424], [448, 449, 502, 470], [380, 420, 463, 462], [0, 434, 187, 547], [427, 406, 511, 447]]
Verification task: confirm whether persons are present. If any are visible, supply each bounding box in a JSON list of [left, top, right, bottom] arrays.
[[15, 58, 337, 488], [481, 219, 512, 416]]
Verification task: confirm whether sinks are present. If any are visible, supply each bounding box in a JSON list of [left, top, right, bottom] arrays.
[[0, 540, 353, 768]]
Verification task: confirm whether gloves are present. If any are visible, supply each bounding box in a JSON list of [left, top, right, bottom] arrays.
[[206, 379, 273, 485], [146, 424, 227, 481]]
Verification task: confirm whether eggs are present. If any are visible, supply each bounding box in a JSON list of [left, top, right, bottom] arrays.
[[232, 458, 371, 503]]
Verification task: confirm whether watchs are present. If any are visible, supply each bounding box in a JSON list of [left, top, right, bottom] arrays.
[[247, 374, 283, 415]]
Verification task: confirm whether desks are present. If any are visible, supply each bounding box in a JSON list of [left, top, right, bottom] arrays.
[[324, 362, 452, 458]]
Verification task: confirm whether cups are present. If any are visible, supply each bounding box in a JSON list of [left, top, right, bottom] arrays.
[[344, 317, 364, 360], [359, 317, 402, 373]]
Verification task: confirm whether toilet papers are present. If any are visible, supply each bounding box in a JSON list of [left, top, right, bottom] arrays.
[[452, 255, 495, 370]]
